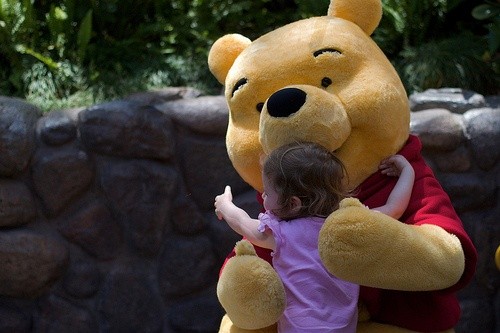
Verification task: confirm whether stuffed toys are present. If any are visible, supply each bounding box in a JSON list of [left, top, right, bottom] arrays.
[[208, 1, 477, 333]]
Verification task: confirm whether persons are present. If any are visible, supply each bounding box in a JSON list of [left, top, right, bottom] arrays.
[[213, 142, 414, 333]]
